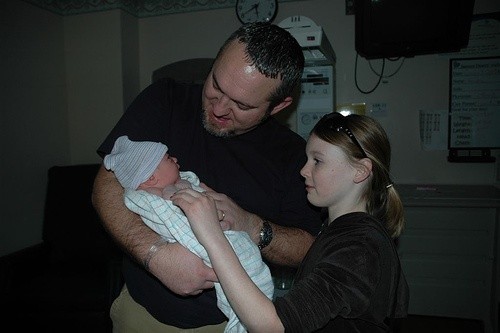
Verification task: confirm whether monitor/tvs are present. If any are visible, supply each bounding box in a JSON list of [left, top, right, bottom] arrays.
[[355, 0, 475, 60]]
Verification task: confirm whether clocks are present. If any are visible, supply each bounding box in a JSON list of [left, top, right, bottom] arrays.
[[235, 1, 279, 25]]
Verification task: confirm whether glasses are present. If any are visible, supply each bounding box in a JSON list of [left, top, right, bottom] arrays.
[[309, 114, 368, 158]]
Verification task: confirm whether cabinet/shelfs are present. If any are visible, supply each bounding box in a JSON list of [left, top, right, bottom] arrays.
[[395, 183, 498, 321]]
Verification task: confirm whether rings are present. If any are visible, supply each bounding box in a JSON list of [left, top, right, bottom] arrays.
[[219, 209, 226, 221]]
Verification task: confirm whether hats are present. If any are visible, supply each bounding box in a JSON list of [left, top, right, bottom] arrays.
[[103, 135, 169, 190]]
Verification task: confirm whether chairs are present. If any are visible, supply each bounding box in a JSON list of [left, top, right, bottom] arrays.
[[0, 164, 115, 333]]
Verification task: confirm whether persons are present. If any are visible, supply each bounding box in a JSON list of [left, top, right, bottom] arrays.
[[90, 20, 329, 333], [170, 111, 410, 333], [103, 135, 275, 333]]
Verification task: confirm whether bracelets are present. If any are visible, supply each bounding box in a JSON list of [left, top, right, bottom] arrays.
[[258, 218, 273, 250], [144, 236, 167, 271]]
